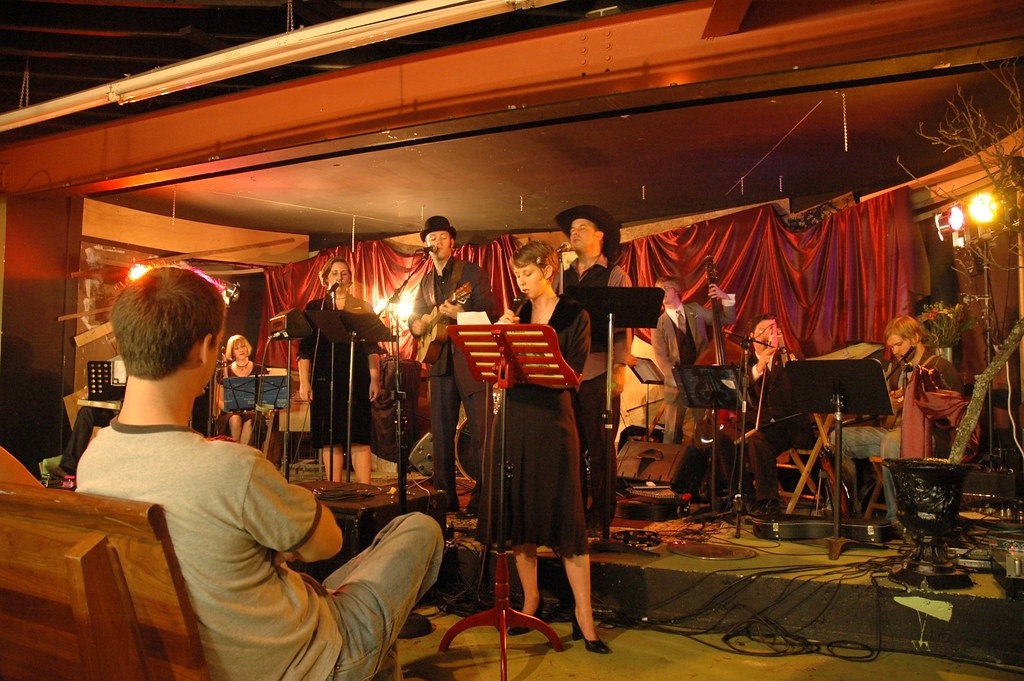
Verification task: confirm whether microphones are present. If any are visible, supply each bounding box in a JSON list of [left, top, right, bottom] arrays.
[[513, 292, 527, 315], [327, 279, 342, 294], [416, 244, 437, 252], [778, 346, 787, 353], [768, 324, 774, 344], [556, 241, 572, 252]]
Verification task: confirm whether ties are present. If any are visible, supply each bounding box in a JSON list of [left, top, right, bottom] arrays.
[[676, 311, 686, 334]]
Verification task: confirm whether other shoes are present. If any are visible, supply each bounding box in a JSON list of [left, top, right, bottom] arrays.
[[746, 505, 780, 525], [464, 508, 478, 517], [676, 497, 691, 517]]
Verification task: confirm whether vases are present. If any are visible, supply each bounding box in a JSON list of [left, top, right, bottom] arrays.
[[933, 347, 952, 364], [880, 458, 981, 591]]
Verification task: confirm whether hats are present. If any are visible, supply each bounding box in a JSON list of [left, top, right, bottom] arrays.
[[419, 216, 457, 242], [556, 205, 621, 257]]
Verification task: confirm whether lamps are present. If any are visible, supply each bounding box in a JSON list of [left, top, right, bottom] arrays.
[[932, 206, 963, 242]]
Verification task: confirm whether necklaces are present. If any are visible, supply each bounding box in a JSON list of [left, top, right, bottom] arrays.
[[237, 362, 249, 370]]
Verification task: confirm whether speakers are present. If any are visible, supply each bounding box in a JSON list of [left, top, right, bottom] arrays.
[[617, 440, 724, 504]]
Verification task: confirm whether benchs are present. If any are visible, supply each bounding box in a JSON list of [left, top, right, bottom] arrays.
[[0, 481, 210, 681]]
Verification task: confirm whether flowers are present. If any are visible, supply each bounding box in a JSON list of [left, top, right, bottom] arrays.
[[922, 300, 975, 349]]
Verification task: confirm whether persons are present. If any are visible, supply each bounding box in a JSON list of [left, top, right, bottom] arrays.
[[651, 276, 737, 445], [59, 387, 125, 489], [462, 242, 611, 655], [407, 216, 494, 514], [295, 257, 383, 486], [829, 315, 964, 532], [741, 315, 825, 517], [215, 335, 269, 447], [551, 204, 634, 538], [73, 266, 446, 681]]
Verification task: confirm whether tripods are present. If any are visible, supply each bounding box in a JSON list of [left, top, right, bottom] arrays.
[[671, 361, 755, 524], [574, 286, 665, 558], [436, 324, 582, 681], [784, 360, 896, 558]]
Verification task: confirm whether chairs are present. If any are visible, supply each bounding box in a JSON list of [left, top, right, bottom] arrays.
[[776, 413, 850, 517], [862, 455, 888, 519]]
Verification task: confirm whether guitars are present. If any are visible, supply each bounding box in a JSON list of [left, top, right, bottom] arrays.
[[417, 282, 474, 364]]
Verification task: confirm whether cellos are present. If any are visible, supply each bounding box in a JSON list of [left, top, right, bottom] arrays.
[[702, 254, 778, 502]]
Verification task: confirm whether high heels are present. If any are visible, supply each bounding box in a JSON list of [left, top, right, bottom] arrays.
[[508, 598, 544, 635], [572, 614, 610, 653]]
